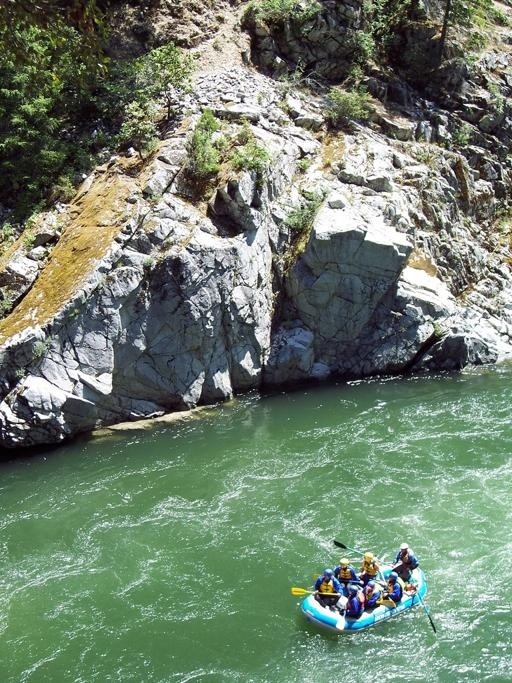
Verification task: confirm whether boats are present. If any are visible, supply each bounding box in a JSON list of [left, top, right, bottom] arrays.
[[299, 553, 426, 636]]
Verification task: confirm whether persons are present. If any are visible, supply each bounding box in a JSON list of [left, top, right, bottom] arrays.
[[315, 543, 419, 619]]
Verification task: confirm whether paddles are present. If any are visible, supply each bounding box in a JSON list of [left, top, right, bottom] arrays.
[[291, 587, 340, 598], [336, 611, 347, 632], [415, 589, 436, 632], [334, 541, 392, 568], [376, 599, 395, 610]]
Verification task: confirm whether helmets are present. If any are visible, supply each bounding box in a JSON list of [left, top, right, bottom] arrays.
[[364, 552, 372, 561], [400, 543, 408, 550], [389, 572, 398, 578], [368, 581, 375, 588], [350, 586, 358, 593], [341, 558, 348, 566], [324, 569, 332, 576]]
